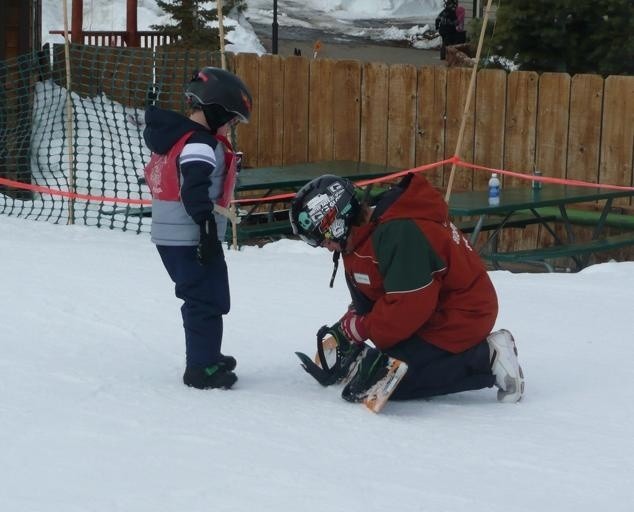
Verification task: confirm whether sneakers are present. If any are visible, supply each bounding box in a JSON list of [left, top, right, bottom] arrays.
[[182, 365, 239, 391], [485, 328, 527, 407]]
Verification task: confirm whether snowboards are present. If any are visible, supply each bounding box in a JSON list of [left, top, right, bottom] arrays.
[[315, 333, 409, 414]]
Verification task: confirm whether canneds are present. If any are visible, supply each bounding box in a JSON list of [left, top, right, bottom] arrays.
[[236, 152, 243, 175], [532, 169, 541, 189]]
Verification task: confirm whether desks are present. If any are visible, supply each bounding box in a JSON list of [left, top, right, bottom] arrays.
[[218, 158, 418, 245], [425, 182, 632, 277]]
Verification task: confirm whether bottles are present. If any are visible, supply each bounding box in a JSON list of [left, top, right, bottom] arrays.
[[488, 172, 501, 205]]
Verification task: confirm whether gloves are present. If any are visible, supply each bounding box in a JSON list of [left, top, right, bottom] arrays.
[[337, 312, 369, 347], [196, 221, 221, 268]]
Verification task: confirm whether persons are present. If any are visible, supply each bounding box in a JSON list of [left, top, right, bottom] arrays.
[[143, 66, 252, 391], [288, 169, 525, 404], [435, 0, 465, 60]]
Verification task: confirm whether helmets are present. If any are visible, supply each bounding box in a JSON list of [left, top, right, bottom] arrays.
[[184, 65, 254, 125], [288, 172, 358, 253]]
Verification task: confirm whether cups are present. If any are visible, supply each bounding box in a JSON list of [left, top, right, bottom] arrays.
[[532, 171, 543, 190], [531, 190, 542, 202], [236, 150, 243, 175]]
